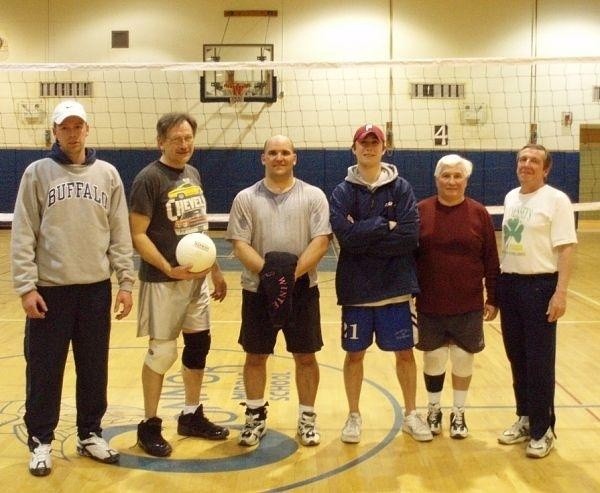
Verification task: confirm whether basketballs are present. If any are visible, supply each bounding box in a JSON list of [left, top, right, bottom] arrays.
[[176, 233, 216, 272]]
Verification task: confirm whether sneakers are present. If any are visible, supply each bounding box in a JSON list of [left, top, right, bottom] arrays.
[[450, 407, 469, 439], [29, 437, 52, 476], [527, 425, 556, 458], [137, 417, 172, 458], [341, 412, 363, 444], [498, 415, 531, 445], [238, 401, 268, 446], [296, 412, 320, 446], [176, 404, 230, 440], [76, 430, 120, 463], [427, 403, 443, 435], [399, 409, 433, 442]]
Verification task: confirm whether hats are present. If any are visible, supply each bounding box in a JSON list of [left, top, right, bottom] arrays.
[[53, 100, 88, 125], [353, 124, 386, 142]]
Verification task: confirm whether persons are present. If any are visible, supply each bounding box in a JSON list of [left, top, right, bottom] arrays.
[[330, 124, 433, 445], [126, 111, 230, 457], [417, 154, 502, 439], [225, 134, 333, 447], [498, 143, 579, 458], [10, 100, 135, 478]]
